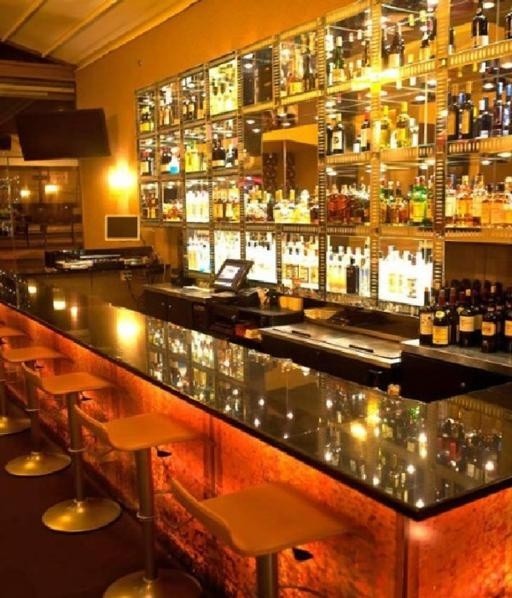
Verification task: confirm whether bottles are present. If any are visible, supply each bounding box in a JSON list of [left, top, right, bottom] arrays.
[[209, 120, 242, 172], [473, 280, 483, 348], [346, 257, 361, 294], [183, 178, 209, 226], [323, 423, 371, 480], [158, 178, 185, 225], [363, 247, 372, 267], [416, 246, 436, 304], [332, 35, 345, 86], [447, 92, 457, 141], [419, 287, 433, 346], [138, 180, 162, 223], [327, 244, 346, 294], [406, 256, 416, 300], [431, 19, 437, 59], [137, 88, 159, 134], [331, 114, 344, 155], [280, 234, 320, 292], [325, 179, 372, 229], [158, 132, 182, 177], [186, 229, 211, 274], [208, 60, 239, 115], [448, 287, 457, 346], [157, 82, 181, 131], [444, 173, 458, 222], [412, 176, 427, 226], [492, 82, 504, 136], [378, 398, 427, 459], [212, 178, 242, 226], [378, 177, 414, 226], [325, 383, 371, 425], [424, 180, 434, 228], [345, 245, 352, 268], [241, 231, 278, 285], [325, 122, 333, 156], [378, 244, 409, 299], [478, 96, 491, 137], [381, 29, 388, 70], [505, 8, 512, 41], [138, 135, 159, 178], [471, 3, 488, 49], [437, 418, 502, 483], [371, 449, 424, 507], [395, 101, 411, 147], [502, 308, 512, 355], [387, 31, 404, 69], [456, 96, 472, 139], [481, 185, 496, 228], [360, 112, 371, 151], [432, 289, 449, 347], [488, 283, 502, 349], [354, 247, 362, 267], [421, 31, 430, 62], [448, 25, 454, 57], [343, 28, 368, 84], [480, 298, 497, 353], [178, 71, 209, 123], [243, 175, 324, 225], [469, 173, 488, 227], [182, 125, 212, 174], [359, 257, 371, 298], [490, 182, 512, 229], [277, 28, 322, 98], [212, 230, 243, 276], [455, 176, 471, 224], [459, 289, 476, 348], [379, 105, 392, 149], [502, 84, 512, 137]]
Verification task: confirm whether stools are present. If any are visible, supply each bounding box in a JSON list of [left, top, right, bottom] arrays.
[[165, 472, 353, 598], [21, 361, 120, 532], [0, 323, 33, 437], [73, 402, 218, 598], [1, 338, 72, 476]]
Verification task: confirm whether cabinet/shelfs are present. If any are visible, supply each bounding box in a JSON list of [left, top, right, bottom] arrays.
[[0, 275, 512, 598], [132, 0, 512, 321]]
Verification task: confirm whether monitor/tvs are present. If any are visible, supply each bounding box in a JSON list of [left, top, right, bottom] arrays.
[[105, 215, 141, 240], [209, 259, 253, 292], [13, 108, 112, 160]]
